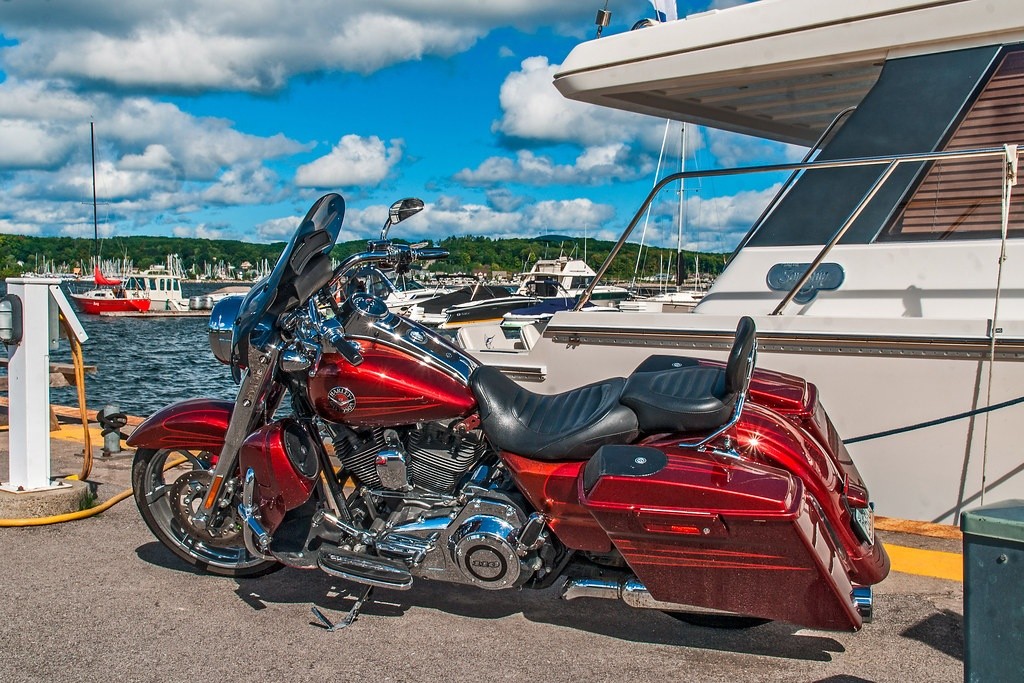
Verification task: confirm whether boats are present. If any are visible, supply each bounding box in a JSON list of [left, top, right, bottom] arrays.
[[19, 118, 278, 317], [319, 118, 727, 353], [453, 0, 1023, 536]]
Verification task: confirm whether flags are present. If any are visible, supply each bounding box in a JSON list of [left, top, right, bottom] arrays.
[[94, 265, 120, 285]]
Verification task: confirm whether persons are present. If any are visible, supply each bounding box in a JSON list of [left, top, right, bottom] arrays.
[[165, 299, 172, 310], [112, 286, 118, 298]]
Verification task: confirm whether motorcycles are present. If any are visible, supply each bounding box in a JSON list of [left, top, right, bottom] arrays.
[[124, 190, 893, 637]]
